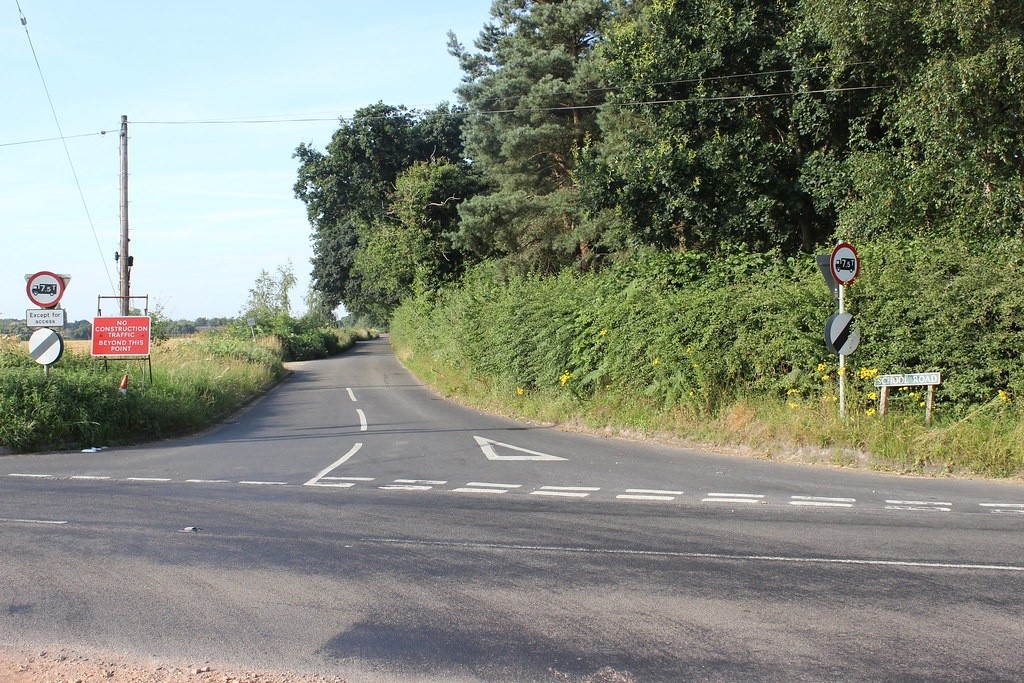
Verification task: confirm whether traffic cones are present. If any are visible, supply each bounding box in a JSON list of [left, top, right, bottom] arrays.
[[116, 375, 131, 397]]
